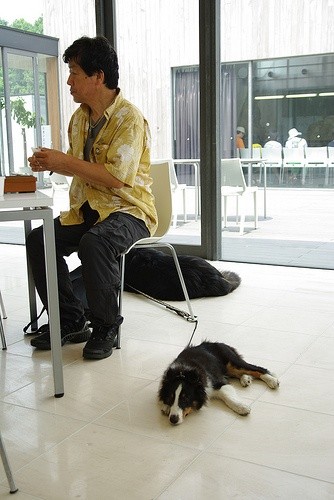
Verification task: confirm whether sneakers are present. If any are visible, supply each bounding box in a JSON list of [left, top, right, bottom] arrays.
[[82, 322, 120, 360], [31, 316, 91, 349]]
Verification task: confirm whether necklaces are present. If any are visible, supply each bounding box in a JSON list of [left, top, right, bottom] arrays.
[[89, 100, 115, 128]]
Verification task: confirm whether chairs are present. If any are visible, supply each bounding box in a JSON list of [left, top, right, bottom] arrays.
[[236, 145, 334, 186], [217, 158, 257, 234], [152, 161, 187, 227], [117, 162, 197, 349]]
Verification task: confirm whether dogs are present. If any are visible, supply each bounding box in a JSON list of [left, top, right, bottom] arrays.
[[157, 340, 279, 426]]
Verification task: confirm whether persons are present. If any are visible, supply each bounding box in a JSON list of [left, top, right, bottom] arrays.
[[285, 128, 307, 180], [27, 35, 158, 361], [235, 126, 245, 149]]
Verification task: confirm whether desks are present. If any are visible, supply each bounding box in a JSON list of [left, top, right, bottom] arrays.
[[170, 158, 268, 223], [0, 176, 65, 398]]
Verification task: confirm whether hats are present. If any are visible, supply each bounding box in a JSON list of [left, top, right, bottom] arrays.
[[287, 128, 302, 139], [236, 126, 245, 133]]
